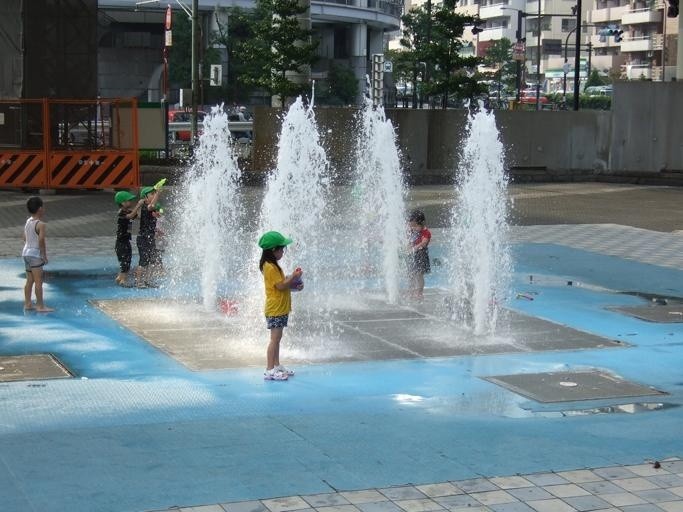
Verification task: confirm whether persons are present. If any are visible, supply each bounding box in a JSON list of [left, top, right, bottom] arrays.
[[399, 209, 434, 303], [21, 196, 55, 313], [113, 191, 147, 289], [230, 100, 239, 122], [473, 91, 489, 110], [135, 184, 165, 290], [258, 229, 304, 382], [236, 105, 251, 140]]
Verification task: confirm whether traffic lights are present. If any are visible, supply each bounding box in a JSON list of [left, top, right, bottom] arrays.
[[606, 28, 623, 42]]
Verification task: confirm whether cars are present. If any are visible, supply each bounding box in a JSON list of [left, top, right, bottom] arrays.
[[169, 110, 209, 142]]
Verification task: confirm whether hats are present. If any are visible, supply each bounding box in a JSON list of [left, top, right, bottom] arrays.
[[140, 187, 157, 197], [115, 191, 136, 203], [258, 231, 292, 250]]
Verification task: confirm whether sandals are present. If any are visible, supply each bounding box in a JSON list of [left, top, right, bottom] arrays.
[[263, 364, 294, 379], [115, 276, 158, 288]]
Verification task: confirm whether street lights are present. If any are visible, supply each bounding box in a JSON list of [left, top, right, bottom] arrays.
[[134, 0, 199, 146], [500, 6, 522, 98]]
